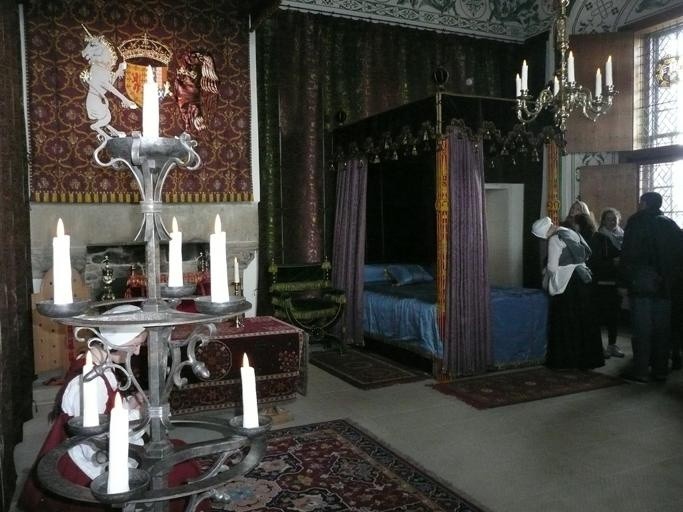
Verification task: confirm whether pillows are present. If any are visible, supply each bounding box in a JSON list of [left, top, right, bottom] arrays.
[[383, 262, 433, 287], [362, 264, 390, 282]]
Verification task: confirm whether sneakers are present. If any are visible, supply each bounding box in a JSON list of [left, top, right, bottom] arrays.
[[602, 345, 624, 358], [581, 360, 605, 369], [649, 373, 673, 384], [618, 373, 649, 385]]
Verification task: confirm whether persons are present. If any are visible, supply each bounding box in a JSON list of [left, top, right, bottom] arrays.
[[562, 199, 590, 228], [14, 304, 230, 512], [529, 215, 604, 370], [566, 214, 621, 277], [595, 205, 626, 359], [616, 192, 678, 383]]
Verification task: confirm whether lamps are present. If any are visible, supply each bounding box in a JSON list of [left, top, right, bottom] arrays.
[[504, 0, 622, 139]]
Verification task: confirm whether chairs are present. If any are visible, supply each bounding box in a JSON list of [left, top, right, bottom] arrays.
[[267, 253, 348, 359]]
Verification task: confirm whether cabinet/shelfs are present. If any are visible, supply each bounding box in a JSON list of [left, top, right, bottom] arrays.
[[107, 312, 308, 424]]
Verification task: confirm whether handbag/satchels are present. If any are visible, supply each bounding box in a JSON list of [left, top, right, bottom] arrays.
[[600, 257, 619, 283]]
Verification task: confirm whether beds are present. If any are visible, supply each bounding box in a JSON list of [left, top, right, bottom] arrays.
[[324, 63, 568, 386]]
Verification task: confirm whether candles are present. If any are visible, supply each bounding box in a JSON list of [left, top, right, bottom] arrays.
[[165, 215, 184, 287], [237, 351, 259, 431], [81, 350, 99, 428], [140, 64, 162, 141], [51, 214, 73, 306], [205, 214, 230, 303], [106, 389, 131, 494], [233, 254, 240, 286]]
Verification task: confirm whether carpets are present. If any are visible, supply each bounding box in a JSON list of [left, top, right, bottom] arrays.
[[303, 344, 429, 394], [423, 359, 632, 414], [5, 415, 496, 509]]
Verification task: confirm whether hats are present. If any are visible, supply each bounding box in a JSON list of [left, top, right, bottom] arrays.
[[570, 200, 590, 217], [99, 304, 146, 346], [531, 216, 554, 240]]
